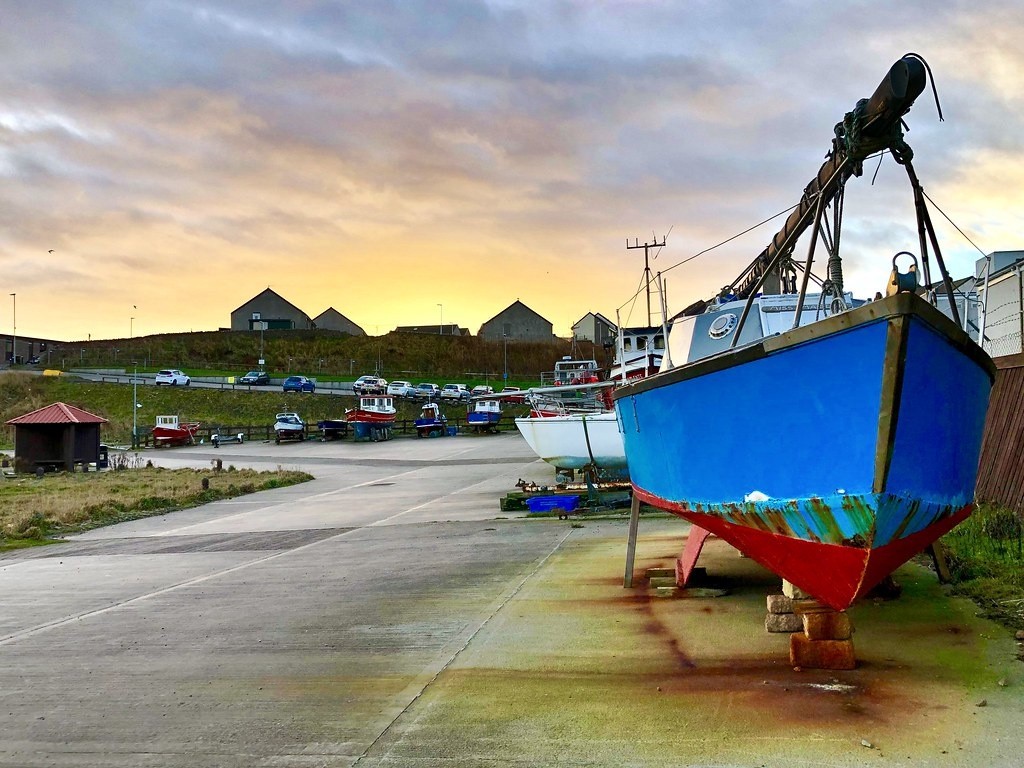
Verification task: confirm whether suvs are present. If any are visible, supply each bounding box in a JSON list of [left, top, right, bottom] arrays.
[[240, 371, 271, 385], [155, 369, 191, 385]]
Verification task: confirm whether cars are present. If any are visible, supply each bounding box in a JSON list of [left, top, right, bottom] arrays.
[[283, 376, 315, 393], [441, 384, 472, 400], [500, 386, 527, 404], [387, 381, 415, 399], [414, 383, 441, 397], [469, 384, 497, 399], [353, 375, 388, 394]]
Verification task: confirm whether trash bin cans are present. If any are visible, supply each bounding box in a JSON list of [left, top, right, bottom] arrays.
[[99, 446, 108, 468]]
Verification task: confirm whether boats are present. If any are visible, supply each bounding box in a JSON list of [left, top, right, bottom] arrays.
[[344, 394, 397, 441], [273, 413, 308, 442], [413, 391, 450, 439], [152, 415, 204, 446], [513, 52, 996, 611], [467, 369, 504, 427], [317, 420, 348, 440]]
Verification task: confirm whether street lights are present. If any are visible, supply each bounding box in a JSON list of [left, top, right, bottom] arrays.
[[130, 317, 135, 338], [10, 293, 16, 363], [131, 361, 139, 435]]
